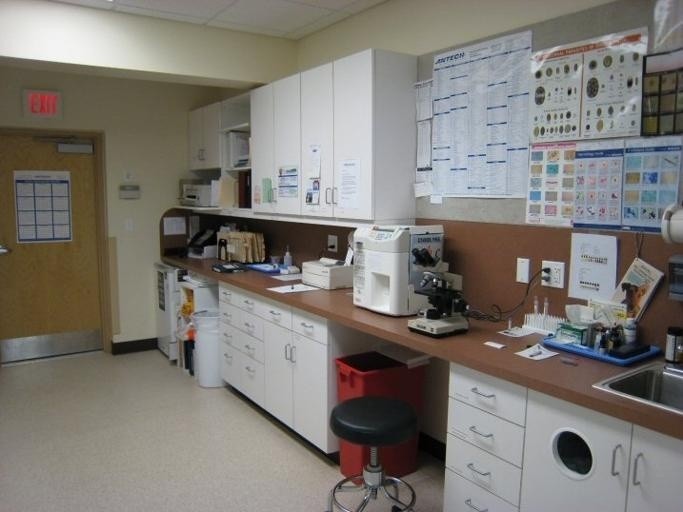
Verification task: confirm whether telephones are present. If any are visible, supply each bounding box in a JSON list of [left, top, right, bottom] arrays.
[[188, 227, 218, 248]]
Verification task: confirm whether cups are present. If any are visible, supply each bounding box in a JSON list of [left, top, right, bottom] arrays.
[[664, 325, 683, 365]]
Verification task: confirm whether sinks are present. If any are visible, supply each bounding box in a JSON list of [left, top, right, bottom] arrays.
[[590, 361, 683, 416]]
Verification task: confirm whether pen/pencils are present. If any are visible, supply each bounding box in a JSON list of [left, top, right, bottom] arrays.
[[291, 282, 293, 290]]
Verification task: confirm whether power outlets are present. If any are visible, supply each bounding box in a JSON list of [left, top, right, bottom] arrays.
[[539, 259, 564, 289]]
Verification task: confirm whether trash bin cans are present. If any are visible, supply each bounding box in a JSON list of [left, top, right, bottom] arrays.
[[191, 309, 227, 387], [332, 352, 425, 485]]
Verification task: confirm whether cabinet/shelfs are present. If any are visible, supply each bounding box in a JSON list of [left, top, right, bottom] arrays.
[[519, 385, 683, 511], [441, 361, 528, 511], [184, 48, 417, 229], [216, 281, 396, 456]]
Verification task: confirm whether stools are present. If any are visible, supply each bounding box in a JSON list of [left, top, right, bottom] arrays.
[[329, 394, 419, 512]]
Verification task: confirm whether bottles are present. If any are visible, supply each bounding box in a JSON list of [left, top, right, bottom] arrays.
[[284, 244, 292, 265], [508, 317, 513, 330], [592, 322, 623, 354], [533, 295, 549, 319], [623, 317, 638, 346]]
